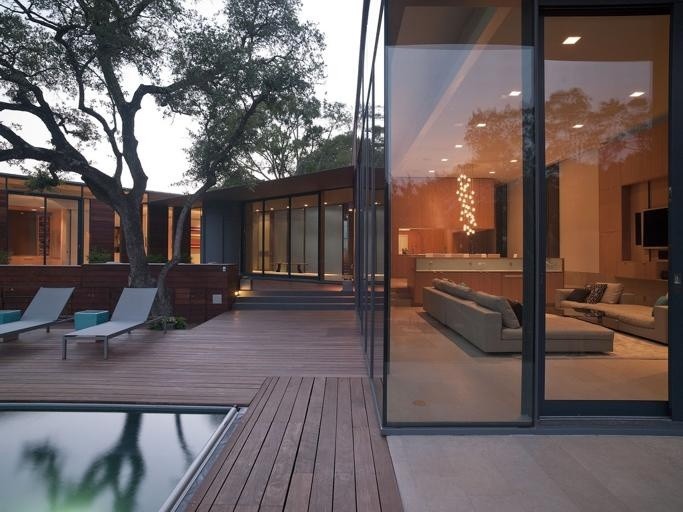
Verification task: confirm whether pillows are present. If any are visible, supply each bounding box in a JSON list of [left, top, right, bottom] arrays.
[[565, 283, 624, 305], [653, 296, 667, 317], [433, 277, 523, 329]]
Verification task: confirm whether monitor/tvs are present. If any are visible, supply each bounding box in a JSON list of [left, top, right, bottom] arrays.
[[641, 206, 669, 250]]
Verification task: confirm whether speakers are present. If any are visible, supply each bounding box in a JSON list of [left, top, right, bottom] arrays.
[[635, 212, 641, 246]]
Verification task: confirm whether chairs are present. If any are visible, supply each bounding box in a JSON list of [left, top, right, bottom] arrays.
[[0, 287, 167, 360]]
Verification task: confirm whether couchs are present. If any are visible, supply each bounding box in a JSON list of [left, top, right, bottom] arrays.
[[555, 281, 668, 345], [423, 278, 614, 354]]
